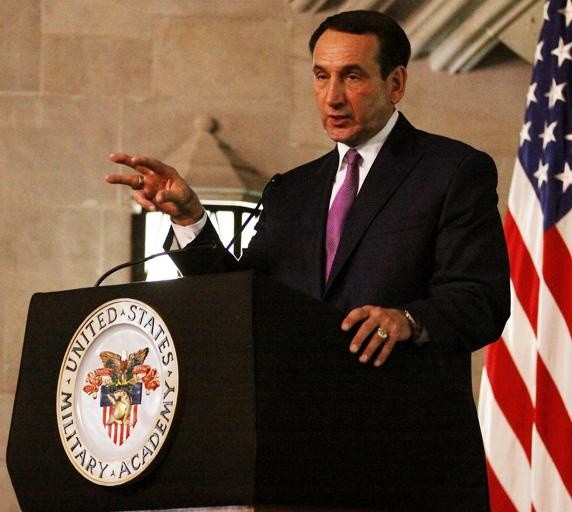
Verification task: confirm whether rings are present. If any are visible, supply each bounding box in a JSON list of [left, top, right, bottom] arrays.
[[377, 327, 388, 341], [138, 174, 145, 189]]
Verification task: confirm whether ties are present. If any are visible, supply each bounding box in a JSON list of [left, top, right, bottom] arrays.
[[325, 148, 362, 289]]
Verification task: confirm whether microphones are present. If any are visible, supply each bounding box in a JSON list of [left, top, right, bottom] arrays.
[[221, 172, 284, 253]]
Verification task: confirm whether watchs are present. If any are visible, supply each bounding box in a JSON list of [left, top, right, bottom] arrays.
[[402, 300, 424, 345]]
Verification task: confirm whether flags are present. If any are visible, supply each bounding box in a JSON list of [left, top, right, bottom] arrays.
[[476, 0, 571, 512]]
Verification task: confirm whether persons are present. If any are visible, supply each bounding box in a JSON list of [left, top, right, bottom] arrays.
[[102, 5, 513, 511]]
